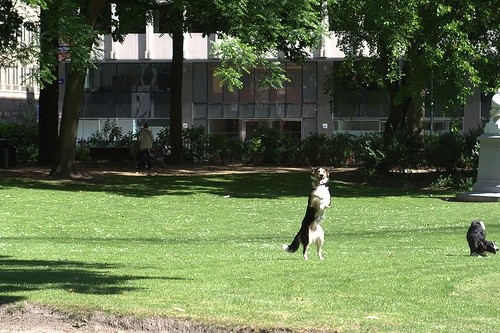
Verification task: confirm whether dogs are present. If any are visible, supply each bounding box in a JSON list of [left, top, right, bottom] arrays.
[[466, 220, 499, 258], [282, 165, 334, 261]]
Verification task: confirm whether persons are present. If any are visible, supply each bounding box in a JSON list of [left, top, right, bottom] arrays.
[[136, 123, 154, 169]]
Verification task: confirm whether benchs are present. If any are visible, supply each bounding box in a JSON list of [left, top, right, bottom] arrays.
[[89, 147, 126, 168]]
